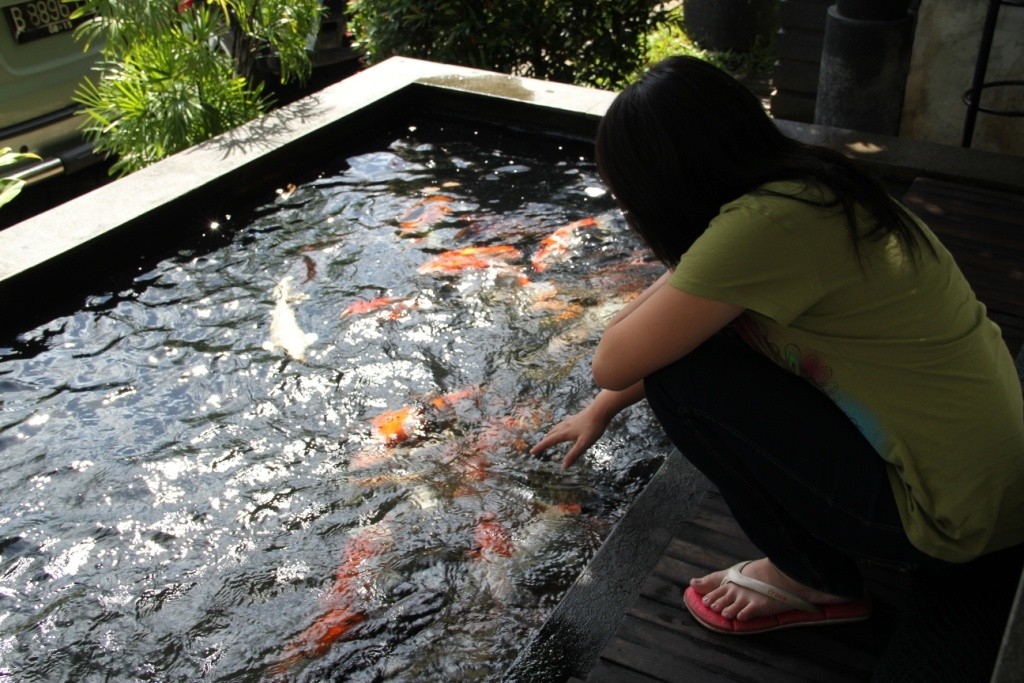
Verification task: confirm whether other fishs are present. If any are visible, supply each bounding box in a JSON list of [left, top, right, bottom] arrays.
[[268, 193, 668, 660]]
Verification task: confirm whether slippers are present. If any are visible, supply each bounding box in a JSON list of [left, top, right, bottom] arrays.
[[682, 560, 876, 634]]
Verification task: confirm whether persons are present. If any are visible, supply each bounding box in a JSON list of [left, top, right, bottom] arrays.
[[530, 56, 1024, 634]]
